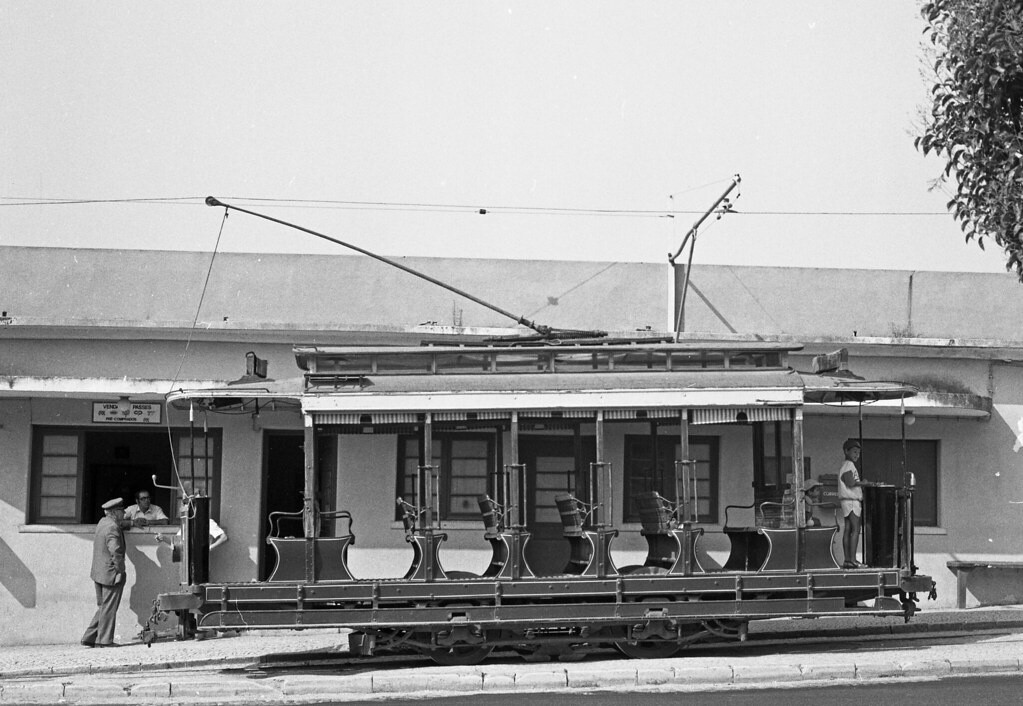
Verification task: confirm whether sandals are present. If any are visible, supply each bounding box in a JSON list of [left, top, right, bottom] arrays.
[[843, 560, 869, 569]]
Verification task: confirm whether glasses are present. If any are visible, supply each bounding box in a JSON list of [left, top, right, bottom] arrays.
[[139, 497, 151, 501], [115, 508, 126, 511]]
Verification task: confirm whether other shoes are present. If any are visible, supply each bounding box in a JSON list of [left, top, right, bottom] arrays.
[[81, 640, 95, 648], [94, 642, 119, 648]]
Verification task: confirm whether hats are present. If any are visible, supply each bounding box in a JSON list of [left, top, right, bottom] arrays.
[[101, 497, 127, 510], [843, 440, 865, 451]]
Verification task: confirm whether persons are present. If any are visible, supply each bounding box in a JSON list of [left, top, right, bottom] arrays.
[[838, 438, 871, 569], [154, 517, 228, 552], [804, 478, 824, 527], [123, 490, 169, 526], [80, 497, 147, 648]]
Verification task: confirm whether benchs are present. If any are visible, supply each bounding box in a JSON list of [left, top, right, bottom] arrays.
[[260, 496, 842, 584]]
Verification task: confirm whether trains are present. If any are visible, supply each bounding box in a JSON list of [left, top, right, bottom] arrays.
[[131, 196, 938, 666]]
